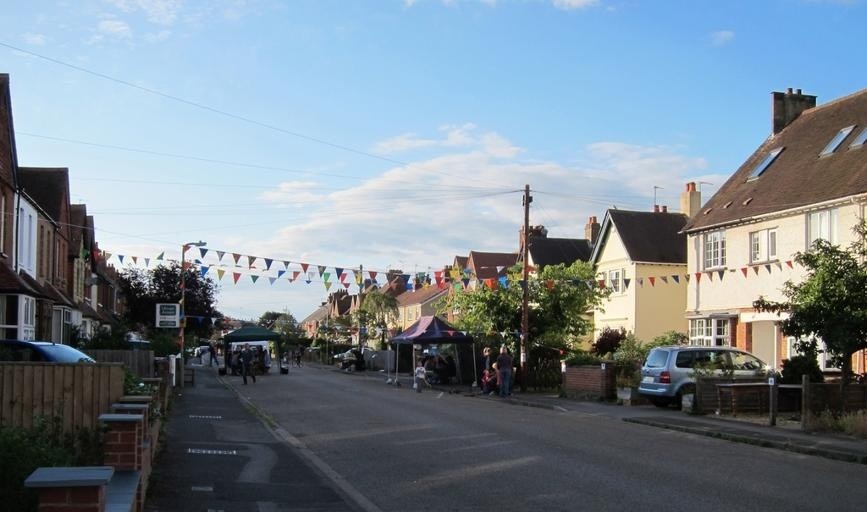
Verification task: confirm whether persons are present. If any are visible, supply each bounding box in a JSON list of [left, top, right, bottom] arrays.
[[477, 344, 513, 399], [414, 350, 456, 391], [196, 342, 304, 385]]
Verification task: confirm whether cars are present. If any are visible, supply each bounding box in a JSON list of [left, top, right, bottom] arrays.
[[334, 348, 375, 360], [0, 339, 96, 364]]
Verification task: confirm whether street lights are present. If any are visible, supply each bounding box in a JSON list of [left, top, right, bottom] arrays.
[[318, 306, 329, 365], [180, 243, 206, 388]]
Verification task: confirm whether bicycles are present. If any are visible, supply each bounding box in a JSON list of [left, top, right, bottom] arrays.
[[292, 360, 304, 368]]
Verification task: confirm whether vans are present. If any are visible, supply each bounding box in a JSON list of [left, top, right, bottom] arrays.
[[638, 346, 779, 411]]
